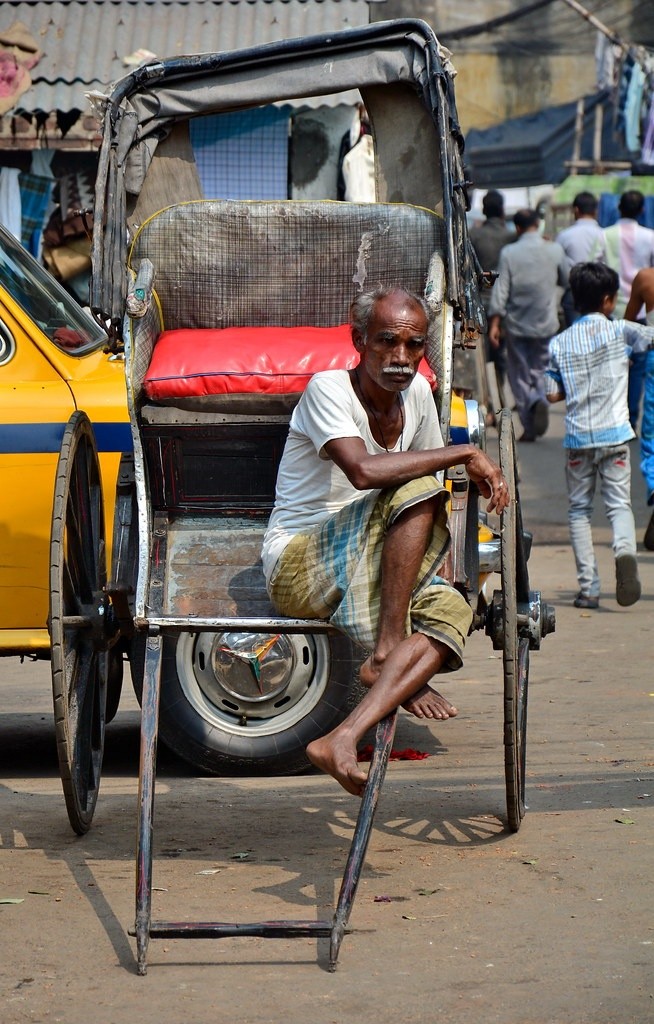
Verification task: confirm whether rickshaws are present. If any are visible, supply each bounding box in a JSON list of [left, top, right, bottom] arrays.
[[40, 13, 562, 976]]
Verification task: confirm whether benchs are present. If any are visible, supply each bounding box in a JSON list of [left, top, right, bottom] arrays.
[[123, 196, 452, 427]]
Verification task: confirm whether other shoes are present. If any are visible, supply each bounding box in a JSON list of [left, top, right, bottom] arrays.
[[644, 510, 654, 550], [547, 393, 565, 402], [616, 553, 641, 606], [574, 592, 599, 608], [533, 399, 548, 436], [518, 435, 535, 443]]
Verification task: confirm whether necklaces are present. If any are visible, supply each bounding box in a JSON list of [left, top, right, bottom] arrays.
[[354, 368, 404, 452]]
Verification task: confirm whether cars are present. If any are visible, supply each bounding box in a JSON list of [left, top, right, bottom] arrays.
[[1, 212, 560, 780]]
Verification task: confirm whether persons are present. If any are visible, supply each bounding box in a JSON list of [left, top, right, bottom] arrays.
[[556, 191, 607, 328], [261, 285, 509, 797], [600, 189, 654, 441], [468, 190, 513, 426], [624, 266, 654, 551], [544, 261, 654, 607], [487, 208, 570, 442]]
